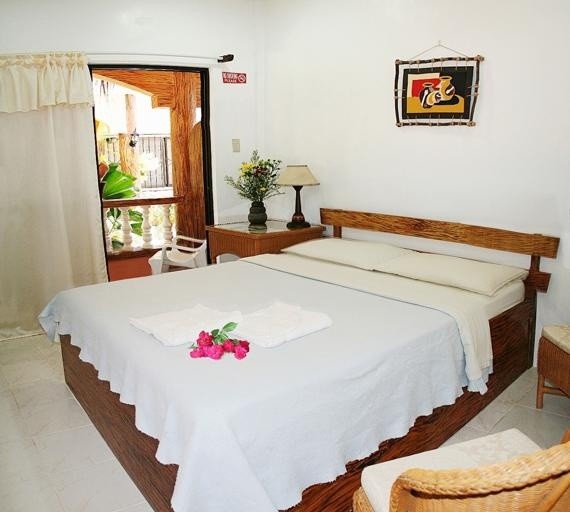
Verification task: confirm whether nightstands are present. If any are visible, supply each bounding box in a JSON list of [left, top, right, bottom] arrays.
[[205, 218, 326, 264]]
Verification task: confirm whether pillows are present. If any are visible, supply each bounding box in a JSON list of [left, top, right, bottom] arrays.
[[281, 237, 529, 297]]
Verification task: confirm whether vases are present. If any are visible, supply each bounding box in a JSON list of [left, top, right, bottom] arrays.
[[248, 201, 267, 229]]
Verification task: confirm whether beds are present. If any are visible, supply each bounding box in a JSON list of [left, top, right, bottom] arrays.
[[38, 207, 560, 512]]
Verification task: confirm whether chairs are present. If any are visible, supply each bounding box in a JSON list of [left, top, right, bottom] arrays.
[[148, 235, 207, 275], [352, 428, 570, 512], [536, 324, 570, 409]]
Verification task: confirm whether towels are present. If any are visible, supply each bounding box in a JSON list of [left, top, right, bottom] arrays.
[[130, 301, 332, 350]]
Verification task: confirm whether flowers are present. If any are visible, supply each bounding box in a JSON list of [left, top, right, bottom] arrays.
[[189, 322, 250, 360], [224, 149, 285, 202]]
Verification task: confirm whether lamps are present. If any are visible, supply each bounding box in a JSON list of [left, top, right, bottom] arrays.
[[273, 164, 320, 228]]
